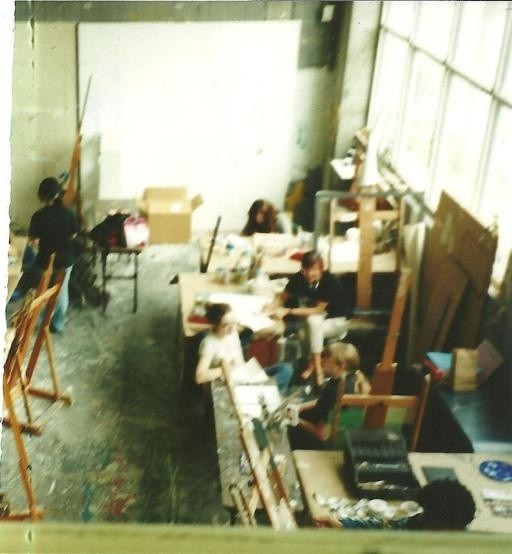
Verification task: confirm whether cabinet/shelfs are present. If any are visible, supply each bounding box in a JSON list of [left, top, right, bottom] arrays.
[[417, 380, 510, 454]]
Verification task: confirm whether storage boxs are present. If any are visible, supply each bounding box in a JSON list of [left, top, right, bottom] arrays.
[[133, 186, 204, 244]]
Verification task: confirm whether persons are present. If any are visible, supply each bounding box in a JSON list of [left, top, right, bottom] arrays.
[[239, 197, 283, 236], [20, 177, 78, 336], [261, 247, 354, 389], [310, 473, 481, 530], [190, 300, 290, 399], [287, 336, 372, 450]]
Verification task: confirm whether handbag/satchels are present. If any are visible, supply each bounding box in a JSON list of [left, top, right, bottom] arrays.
[[88, 209, 149, 248], [450, 347, 479, 392]]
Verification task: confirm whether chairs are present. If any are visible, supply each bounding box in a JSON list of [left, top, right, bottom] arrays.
[[331, 394, 420, 447]]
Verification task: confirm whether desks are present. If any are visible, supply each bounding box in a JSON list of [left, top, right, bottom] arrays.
[[182, 271, 287, 339], [292, 449, 512, 530], [212, 371, 305, 526], [202, 232, 398, 276], [97, 207, 143, 314]]
